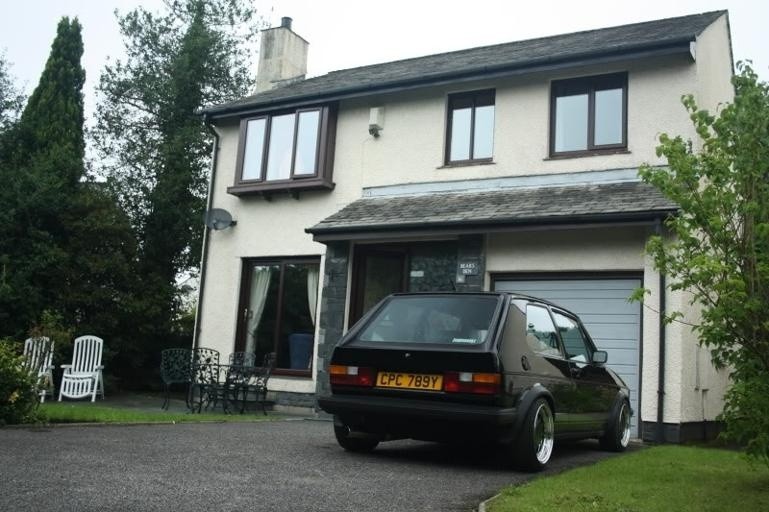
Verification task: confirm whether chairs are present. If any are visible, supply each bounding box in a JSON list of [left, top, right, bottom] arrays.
[[57, 334, 107, 404], [21, 335, 57, 403], [155, 344, 275, 415]]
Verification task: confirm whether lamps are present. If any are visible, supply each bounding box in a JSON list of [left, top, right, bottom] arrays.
[[201, 208, 238, 232]]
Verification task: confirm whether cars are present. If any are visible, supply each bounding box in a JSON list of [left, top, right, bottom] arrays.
[[313, 289, 633, 471]]
